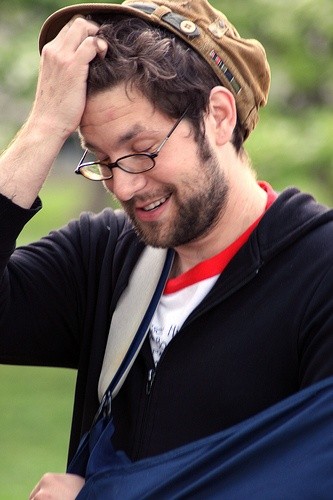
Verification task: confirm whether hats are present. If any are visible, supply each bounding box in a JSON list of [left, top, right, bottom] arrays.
[[38, 1, 271, 142]]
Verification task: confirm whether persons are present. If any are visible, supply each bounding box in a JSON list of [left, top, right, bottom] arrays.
[[0, 0, 333, 500]]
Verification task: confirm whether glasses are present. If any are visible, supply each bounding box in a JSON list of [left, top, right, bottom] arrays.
[[73, 105, 191, 182]]
